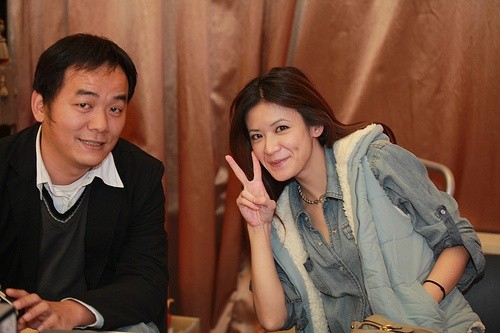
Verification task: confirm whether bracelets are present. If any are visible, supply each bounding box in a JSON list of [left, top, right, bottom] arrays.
[[423, 280, 445, 300]]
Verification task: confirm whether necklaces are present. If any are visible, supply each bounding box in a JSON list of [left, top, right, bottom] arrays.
[[298, 183, 326, 205]]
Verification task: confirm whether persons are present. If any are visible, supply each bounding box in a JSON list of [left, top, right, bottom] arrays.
[[0, 34, 168, 333], [227, 65, 490, 333]]
